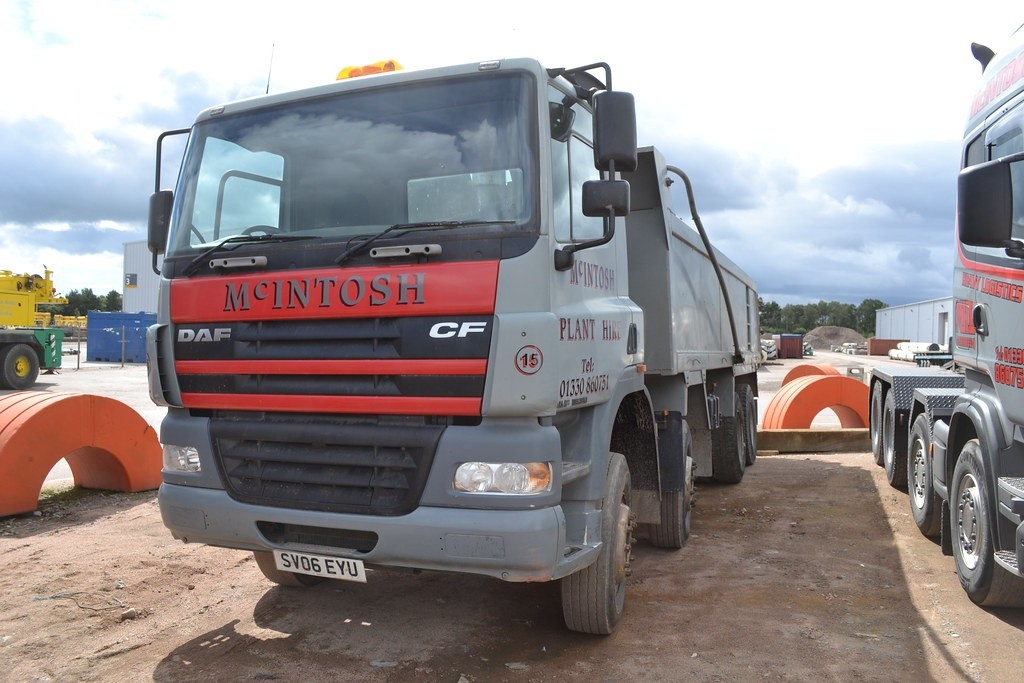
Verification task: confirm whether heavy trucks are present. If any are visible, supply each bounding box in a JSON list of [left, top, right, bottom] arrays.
[[141, 57, 760, 637], [866, 21, 1024, 609]]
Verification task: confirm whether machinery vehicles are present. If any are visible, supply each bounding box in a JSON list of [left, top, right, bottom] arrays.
[[0, 267, 70, 395]]
